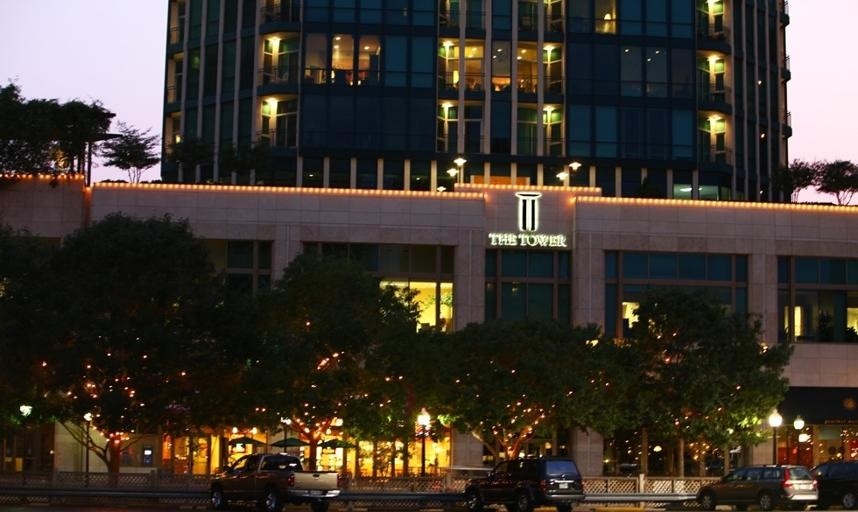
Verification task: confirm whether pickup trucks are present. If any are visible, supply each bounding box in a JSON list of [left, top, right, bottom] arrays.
[[206, 451, 343, 512]]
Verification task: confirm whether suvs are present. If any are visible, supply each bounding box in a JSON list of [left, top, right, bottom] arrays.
[[809, 461, 858, 510], [694, 463, 822, 512], [462, 454, 586, 512]]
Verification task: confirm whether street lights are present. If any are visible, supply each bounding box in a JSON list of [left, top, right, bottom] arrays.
[[767, 409, 784, 465], [83, 412, 93, 487], [793, 413, 806, 465], [416, 406, 432, 476], [279, 416, 292, 452]]
